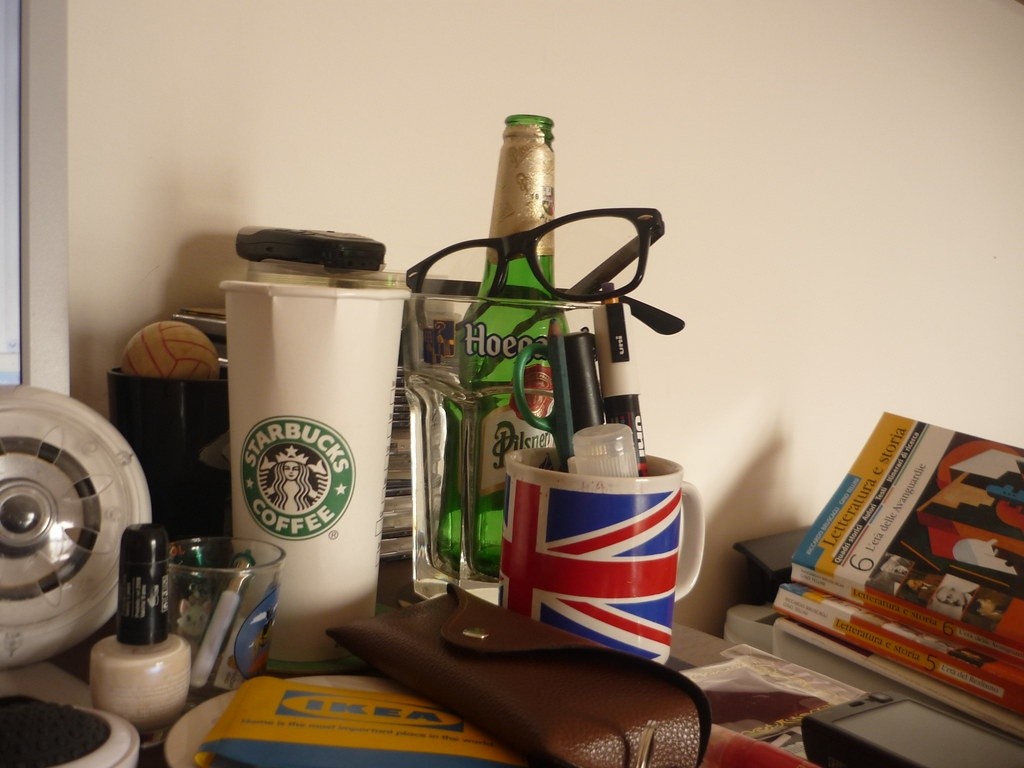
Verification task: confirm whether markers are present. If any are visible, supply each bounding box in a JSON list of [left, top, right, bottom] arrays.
[[565, 301, 649, 479]]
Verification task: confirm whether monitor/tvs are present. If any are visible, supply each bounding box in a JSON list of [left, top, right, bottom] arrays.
[[0, 0, 71, 401]]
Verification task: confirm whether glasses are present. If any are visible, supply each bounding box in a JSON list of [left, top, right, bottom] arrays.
[[407, 208, 687, 336]]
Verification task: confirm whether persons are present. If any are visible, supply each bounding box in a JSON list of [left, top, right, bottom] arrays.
[[936, 585, 979, 611], [905, 574, 942, 601], [869, 552, 908, 581], [972, 596, 1007, 631]]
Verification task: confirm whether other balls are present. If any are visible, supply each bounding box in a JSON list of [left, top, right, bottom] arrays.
[[120, 320, 220, 382]]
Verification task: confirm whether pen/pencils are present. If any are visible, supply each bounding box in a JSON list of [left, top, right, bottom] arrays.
[[548, 317, 575, 458]]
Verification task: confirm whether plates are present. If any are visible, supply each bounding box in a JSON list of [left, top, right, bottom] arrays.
[[165, 674, 418, 768]]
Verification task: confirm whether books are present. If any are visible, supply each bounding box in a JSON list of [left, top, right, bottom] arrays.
[[772, 409, 1024, 721], [171, 306, 417, 562]]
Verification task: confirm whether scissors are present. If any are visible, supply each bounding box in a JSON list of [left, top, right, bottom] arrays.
[[513, 341, 559, 454]]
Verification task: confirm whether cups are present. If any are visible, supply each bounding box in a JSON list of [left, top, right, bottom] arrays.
[[497, 447, 705, 668], [170, 538, 286, 712], [395, 285, 608, 606], [217, 278, 413, 684], [105, 370, 231, 545]]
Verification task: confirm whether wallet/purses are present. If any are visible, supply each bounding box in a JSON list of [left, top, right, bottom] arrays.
[[325, 583, 711, 768]]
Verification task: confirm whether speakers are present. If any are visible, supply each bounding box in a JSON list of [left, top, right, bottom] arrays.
[[0, 385, 153, 669]]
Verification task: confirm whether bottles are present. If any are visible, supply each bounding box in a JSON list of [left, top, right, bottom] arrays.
[[437, 115, 562, 585]]
